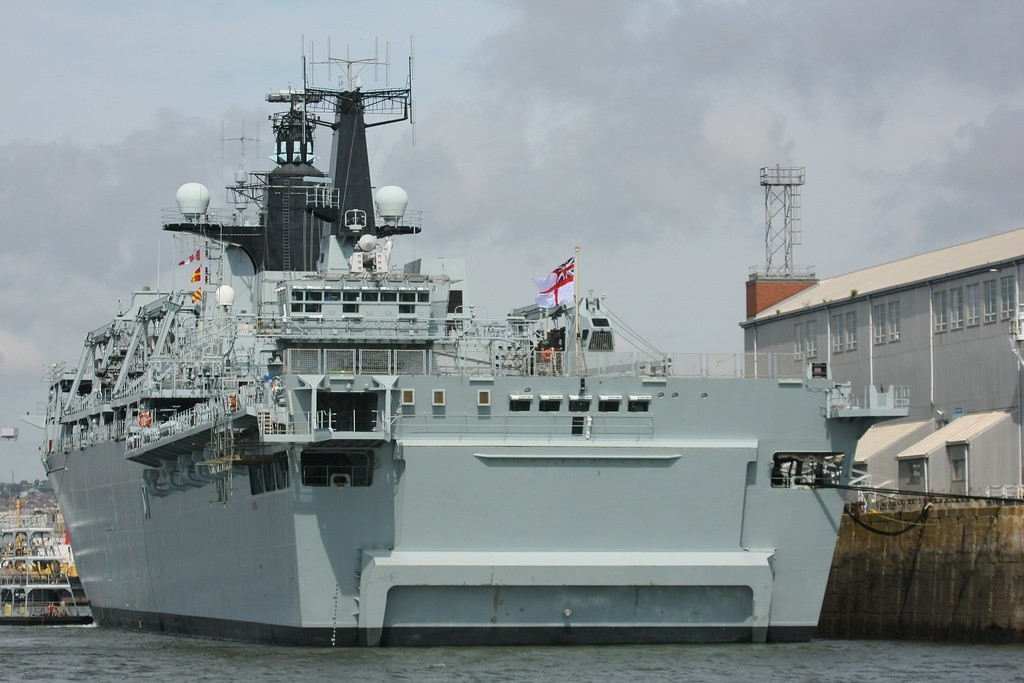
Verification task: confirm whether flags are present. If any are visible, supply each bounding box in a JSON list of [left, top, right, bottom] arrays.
[[191, 287, 202, 304], [191, 267, 200, 282], [178, 249, 200, 265], [534, 257, 574, 308]]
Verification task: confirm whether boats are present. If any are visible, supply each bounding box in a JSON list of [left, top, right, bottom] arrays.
[[18, 33, 911, 648], [0, 470, 92, 625]]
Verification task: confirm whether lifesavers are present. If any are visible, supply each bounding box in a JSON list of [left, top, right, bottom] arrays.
[[543, 349, 552, 360], [138, 412, 151, 427]]
